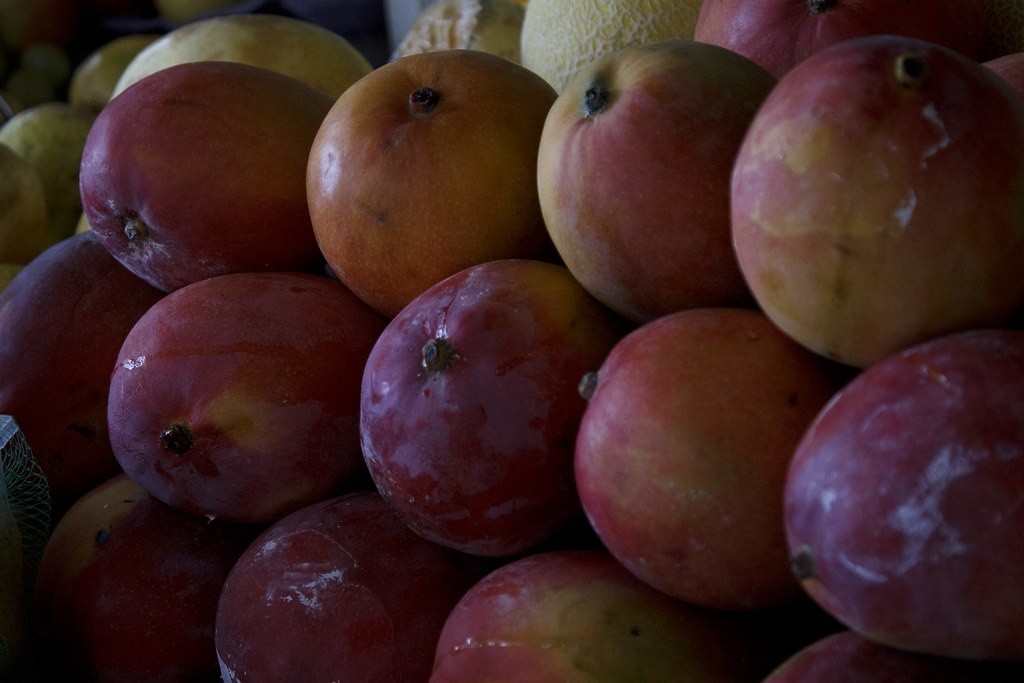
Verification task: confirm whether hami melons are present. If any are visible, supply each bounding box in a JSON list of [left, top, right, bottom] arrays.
[[519, 0, 705, 97]]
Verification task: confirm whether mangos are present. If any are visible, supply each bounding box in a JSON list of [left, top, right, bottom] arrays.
[[0, 0, 1024, 683]]
